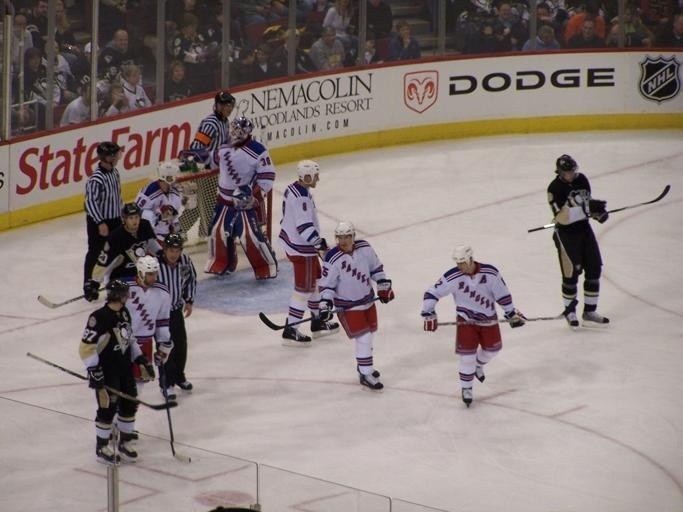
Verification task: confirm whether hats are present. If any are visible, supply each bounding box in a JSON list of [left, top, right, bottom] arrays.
[[85, 41, 100, 54]]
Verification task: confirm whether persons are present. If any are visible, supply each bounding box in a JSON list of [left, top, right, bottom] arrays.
[[420, 244, 526, 404], [111, 255, 176, 441], [82, 140, 123, 286], [177, 90, 235, 240], [82, 201, 156, 302], [78, 280, 154, 466], [133, 159, 185, 250], [446, 1, 682, 53], [150, 233, 198, 400], [202, 117, 280, 280], [546, 153, 609, 327], [316, 220, 394, 391], [276, 158, 340, 343], [5, 2, 420, 136]]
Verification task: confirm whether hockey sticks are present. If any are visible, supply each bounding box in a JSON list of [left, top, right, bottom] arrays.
[[159, 361, 191, 464], [437, 300, 579, 326], [529, 185, 671, 234], [26, 352, 178, 410], [259, 297, 381, 330], [38, 286, 108, 309]]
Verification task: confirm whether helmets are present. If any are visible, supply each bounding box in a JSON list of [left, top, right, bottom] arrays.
[[136, 255, 159, 281], [165, 232, 183, 249], [97, 141, 120, 158], [555, 154, 577, 174], [215, 91, 236, 106], [121, 202, 140, 215], [157, 162, 179, 185], [296, 159, 320, 182], [334, 221, 356, 236], [106, 280, 129, 303], [453, 245, 473, 264], [231, 117, 253, 138]]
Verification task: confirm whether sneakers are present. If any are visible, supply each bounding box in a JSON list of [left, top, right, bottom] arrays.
[[161, 387, 176, 400], [462, 387, 472, 403], [566, 311, 580, 327], [476, 366, 485, 381], [582, 311, 609, 324], [357, 366, 384, 389], [178, 381, 192, 390]]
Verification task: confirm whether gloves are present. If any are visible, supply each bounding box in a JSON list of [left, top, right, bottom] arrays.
[[154, 341, 175, 366], [135, 354, 155, 381], [88, 365, 105, 391], [318, 300, 333, 322], [582, 198, 609, 224], [422, 311, 439, 332], [83, 278, 99, 302], [314, 238, 328, 259], [504, 307, 526, 328], [376, 279, 394, 303]]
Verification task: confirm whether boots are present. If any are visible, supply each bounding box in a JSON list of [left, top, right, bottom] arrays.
[[118, 430, 139, 458], [311, 312, 339, 332], [95, 433, 121, 464], [282, 318, 311, 342]]
